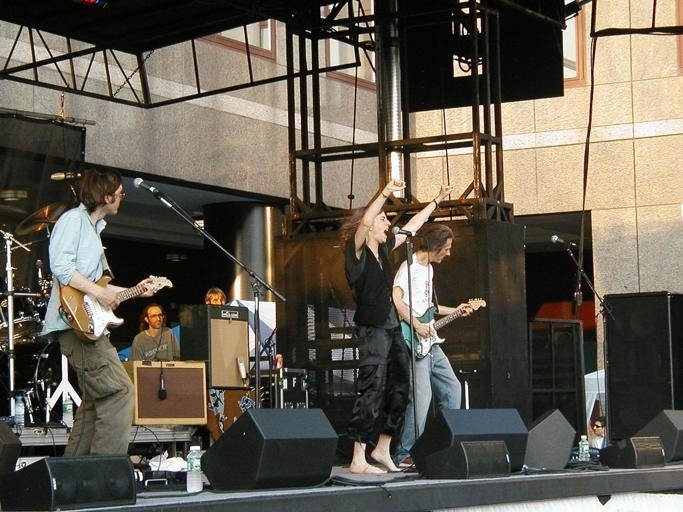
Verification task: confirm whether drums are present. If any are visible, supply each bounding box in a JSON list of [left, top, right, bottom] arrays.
[[0, 286, 41, 344], [15, 352, 54, 386]]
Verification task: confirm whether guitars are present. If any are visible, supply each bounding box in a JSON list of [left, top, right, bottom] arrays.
[[59, 274, 173, 345], [400, 297, 487, 360]]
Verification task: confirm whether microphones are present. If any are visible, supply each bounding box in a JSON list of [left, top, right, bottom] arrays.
[[156, 370, 169, 402], [551, 235, 577, 248], [51, 171, 84, 183], [237, 356, 247, 388], [391, 226, 418, 238], [133, 179, 159, 196]]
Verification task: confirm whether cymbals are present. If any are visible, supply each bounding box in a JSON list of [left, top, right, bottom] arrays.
[[0, 204, 29, 216], [15, 198, 68, 236]]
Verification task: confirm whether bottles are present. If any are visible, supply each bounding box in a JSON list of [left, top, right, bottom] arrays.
[[187, 446, 203, 492], [579, 435, 590, 462], [15, 396, 25, 426], [63, 396, 73, 428]]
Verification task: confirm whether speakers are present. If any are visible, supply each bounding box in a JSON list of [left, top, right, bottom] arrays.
[[201, 408, 337, 490], [16, 454, 135, 511], [124, 361, 207, 425], [421, 440, 511, 480], [603, 292, 679, 451], [599, 437, 668, 469], [397, 223, 531, 408], [409, 409, 529, 473], [631, 407, 683, 461], [527, 407, 577, 474], [180, 304, 250, 390]]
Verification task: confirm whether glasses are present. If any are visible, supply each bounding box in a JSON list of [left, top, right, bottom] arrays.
[[113, 193, 126, 199], [147, 314, 165, 320], [594, 423, 605, 428]]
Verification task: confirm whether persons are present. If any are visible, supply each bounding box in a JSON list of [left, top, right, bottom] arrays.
[[205, 288, 227, 305], [132, 303, 180, 362], [592, 416, 605, 451], [389, 224, 474, 469], [339, 178, 456, 472], [41, 171, 154, 457]]
